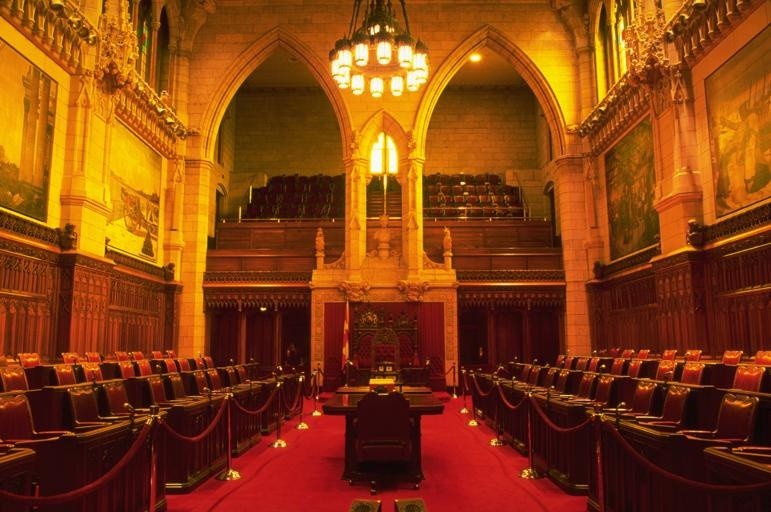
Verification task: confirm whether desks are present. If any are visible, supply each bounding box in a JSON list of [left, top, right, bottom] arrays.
[[338, 384, 432, 394], [323, 392, 445, 483]]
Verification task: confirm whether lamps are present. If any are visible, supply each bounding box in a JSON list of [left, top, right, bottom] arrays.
[[330, 0, 431, 97]]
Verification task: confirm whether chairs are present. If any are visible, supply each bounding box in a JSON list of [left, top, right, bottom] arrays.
[[243, 169, 527, 220], [346, 393, 423, 495], [1, 346, 303, 512], [462, 345, 771, 512], [370, 326, 404, 394]]
